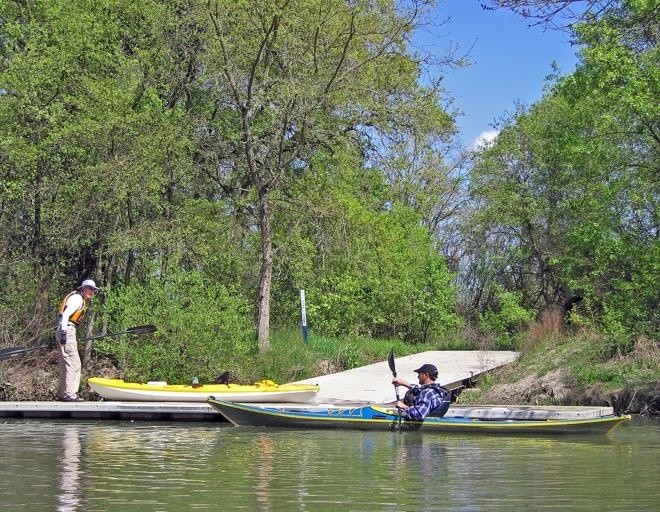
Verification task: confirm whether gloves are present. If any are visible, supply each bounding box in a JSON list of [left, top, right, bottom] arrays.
[[60, 332, 67, 345]]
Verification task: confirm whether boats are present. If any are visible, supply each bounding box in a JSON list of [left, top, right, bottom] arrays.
[[203, 394, 634, 435], [87, 376, 322, 405]]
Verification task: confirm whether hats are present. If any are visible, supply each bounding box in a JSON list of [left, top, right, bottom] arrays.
[[413, 364, 438, 379], [76, 279, 100, 293]]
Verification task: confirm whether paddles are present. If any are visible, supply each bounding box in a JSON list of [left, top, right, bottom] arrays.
[[388, 346, 402, 434], [0, 325, 157, 360]]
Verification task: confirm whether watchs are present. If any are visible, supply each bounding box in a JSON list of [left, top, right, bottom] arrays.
[[404, 405, 409, 410]]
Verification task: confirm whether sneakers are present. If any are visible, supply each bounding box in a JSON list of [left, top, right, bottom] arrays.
[[54, 394, 85, 402]]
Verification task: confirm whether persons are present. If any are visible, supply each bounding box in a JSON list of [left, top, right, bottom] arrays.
[[392, 363, 448, 421], [55, 279, 98, 401]]
